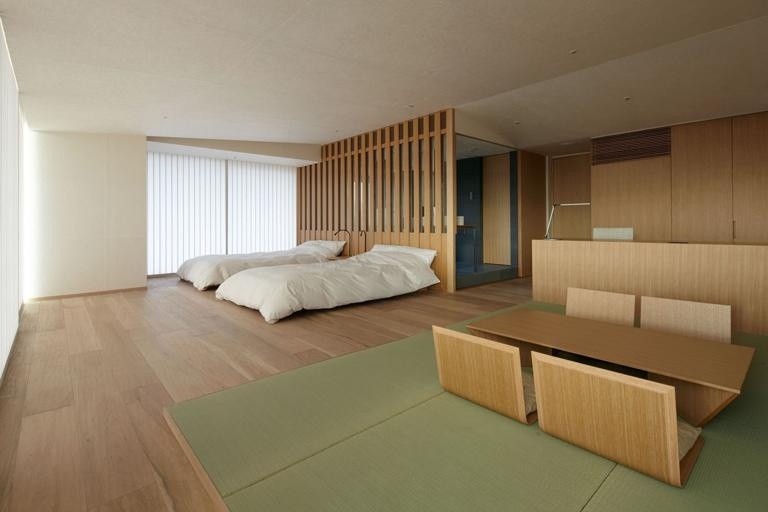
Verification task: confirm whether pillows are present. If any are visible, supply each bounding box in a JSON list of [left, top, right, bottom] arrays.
[[370, 243, 436, 266], [297, 240, 347, 256]]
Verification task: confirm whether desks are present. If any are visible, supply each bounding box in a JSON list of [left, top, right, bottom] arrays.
[[465, 306, 758, 427]]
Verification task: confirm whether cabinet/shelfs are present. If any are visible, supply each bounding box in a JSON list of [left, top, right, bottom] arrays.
[[669, 111, 768, 245], [589, 153, 672, 242]]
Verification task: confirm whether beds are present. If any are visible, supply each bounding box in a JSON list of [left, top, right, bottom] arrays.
[[173, 244, 352, 293], [214, 250, 440, 325]]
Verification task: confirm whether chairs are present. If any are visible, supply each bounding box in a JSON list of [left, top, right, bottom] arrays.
[[563, 286, 635, 328], [638, 294, 733, 342], [429, 324, 539, 428], [529, 349, 704, 488]]
